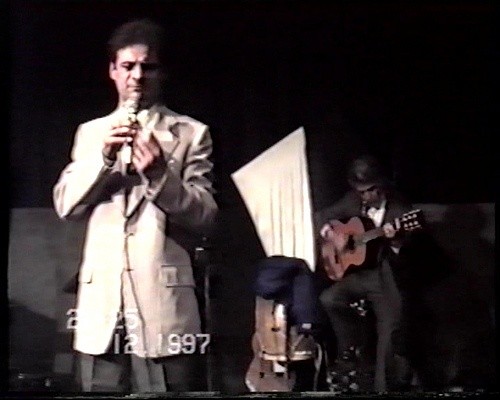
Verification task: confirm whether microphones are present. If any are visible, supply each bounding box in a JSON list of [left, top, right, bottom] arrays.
[[124, 99, 142, 163]]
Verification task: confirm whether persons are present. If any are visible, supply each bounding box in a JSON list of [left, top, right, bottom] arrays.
[[318, 158, 429, 392], [53, 21, 218, 392]]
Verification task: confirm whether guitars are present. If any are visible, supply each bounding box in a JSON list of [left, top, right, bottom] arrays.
[[320, 207, 429, 281]]
